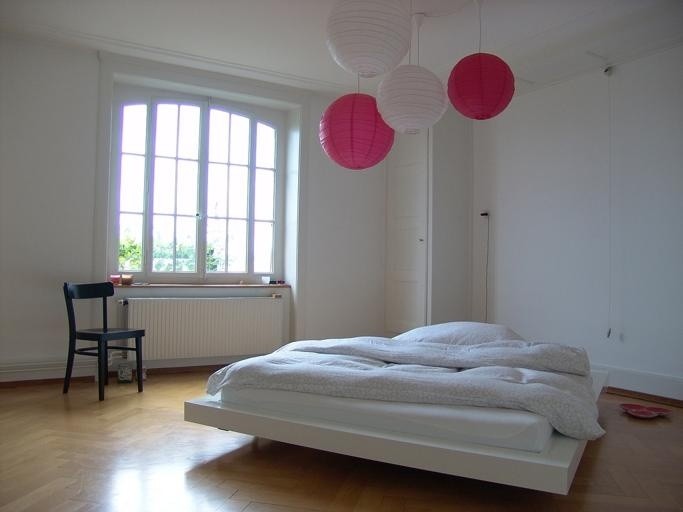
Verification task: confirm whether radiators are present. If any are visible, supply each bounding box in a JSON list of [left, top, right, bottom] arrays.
[[118, 294, 286, 363]]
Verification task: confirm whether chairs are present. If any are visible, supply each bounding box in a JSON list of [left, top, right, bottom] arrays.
[[60, 280, 146, 403]]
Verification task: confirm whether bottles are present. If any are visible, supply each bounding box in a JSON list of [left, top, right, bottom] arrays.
[[116, 363, 147, 384]]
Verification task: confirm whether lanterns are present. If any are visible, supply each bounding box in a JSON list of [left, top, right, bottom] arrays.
[[322, 0, 412, 82], [373, 63, 448, 135], [316, 92, 397, 172], [445, 51, 515, 121]]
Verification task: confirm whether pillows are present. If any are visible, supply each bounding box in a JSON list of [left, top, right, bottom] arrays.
[[387, 319, 528, 346]]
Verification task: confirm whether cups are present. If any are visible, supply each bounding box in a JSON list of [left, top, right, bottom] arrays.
[[109, 274, 133, 285], [261, 276, 271, 285]]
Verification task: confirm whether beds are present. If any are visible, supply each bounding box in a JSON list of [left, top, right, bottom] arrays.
[[183, 335, 609, 499]]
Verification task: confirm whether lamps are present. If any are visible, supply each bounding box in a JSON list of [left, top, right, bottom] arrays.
[[316, 0, 517, 171]]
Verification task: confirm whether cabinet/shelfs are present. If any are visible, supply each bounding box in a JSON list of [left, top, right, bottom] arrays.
[[380, 99, 475, 339]]
